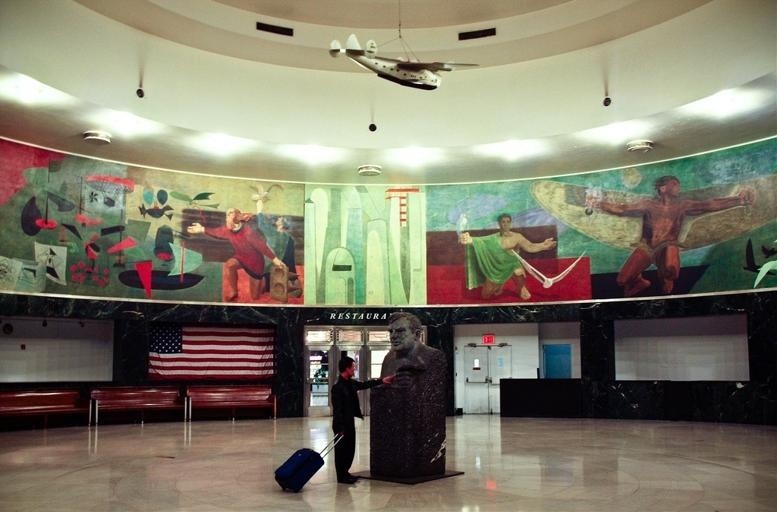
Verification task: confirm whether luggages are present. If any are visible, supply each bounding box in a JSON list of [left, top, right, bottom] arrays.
[[273, 433, 344, 492]]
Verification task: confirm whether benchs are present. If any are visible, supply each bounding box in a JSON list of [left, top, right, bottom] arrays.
[[0, 383, 278, 429]]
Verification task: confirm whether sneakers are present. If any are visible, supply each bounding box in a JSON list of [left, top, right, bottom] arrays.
[[335, 470, 359, 485]]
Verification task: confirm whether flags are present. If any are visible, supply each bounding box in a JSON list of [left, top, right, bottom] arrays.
[[144, 317, 279, 385]]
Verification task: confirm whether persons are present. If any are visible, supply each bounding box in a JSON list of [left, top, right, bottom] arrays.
[[375, 309, 449, 388], [184, 206, 288, 303], [330, 354, 396, 485], [582, 174, 755, 297], [456, 212, 560, 303], [255, 198, 298, 299]]
